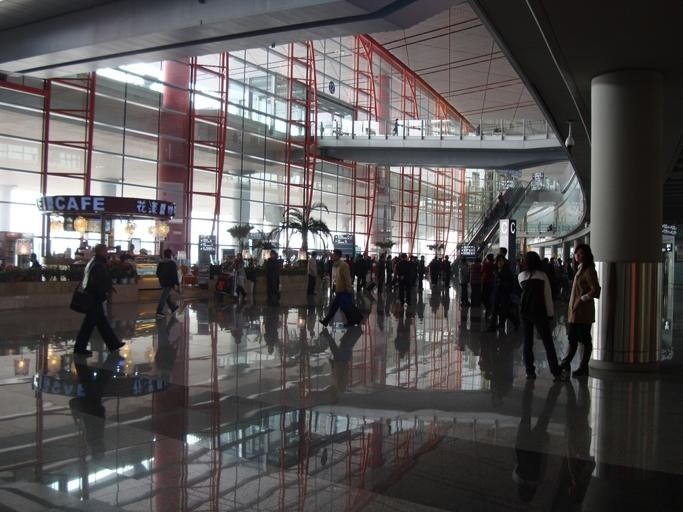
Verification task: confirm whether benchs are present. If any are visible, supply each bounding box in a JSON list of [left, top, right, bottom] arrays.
[[210, 274, 244, 307]]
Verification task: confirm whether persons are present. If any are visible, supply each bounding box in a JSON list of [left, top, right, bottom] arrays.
[[70, 244, 125, 357], [152, 312, 180, 380], [476, 124, 480, 136], [560, 378, 597, 511], [74, 241, 148, 259], [154, 249, 181, 318], [392, 118, 401, 136], [70, 351, 124, 461], [232, 287, 520, 412], [27, 253, 42, 270], [560, 245, 602, 377], [497, 191, 506, 207], [514, 250, 564, 384], [224, 244, 577, 329], [510, 376, 565, 502]]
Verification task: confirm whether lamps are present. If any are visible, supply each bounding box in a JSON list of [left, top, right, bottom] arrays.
[[177, 250, 186, 266], [153, 219, 169, 239], [73, 214, 87, 234], [12, 348, 78, 378]]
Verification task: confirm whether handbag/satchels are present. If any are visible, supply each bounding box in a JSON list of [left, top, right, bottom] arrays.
[[69, 289, 98, 314]]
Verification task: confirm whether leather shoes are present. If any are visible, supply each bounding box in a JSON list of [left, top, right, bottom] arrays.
[[73, 347, 92, 355], [110, 342, 125, 351]]
